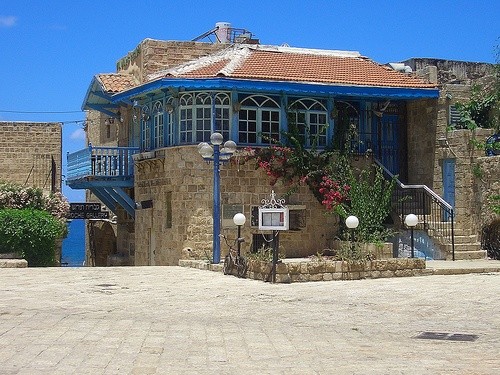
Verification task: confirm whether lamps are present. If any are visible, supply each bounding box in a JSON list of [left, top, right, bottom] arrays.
[[104, 108, 138, 126], [133, 199, 153, 210]]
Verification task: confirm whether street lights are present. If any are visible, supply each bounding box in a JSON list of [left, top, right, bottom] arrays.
[[233, 213, 247, 256], [198, 133, 237, 263], [346, 216, 360, 259], [405, 214, 419, 258]]
